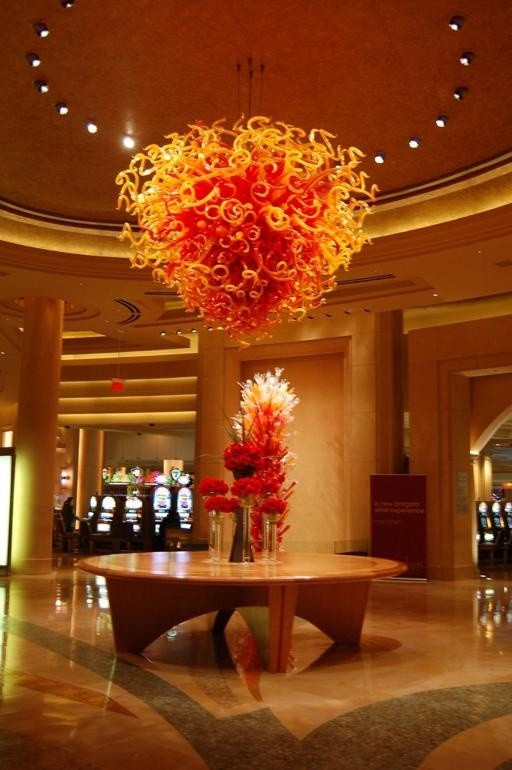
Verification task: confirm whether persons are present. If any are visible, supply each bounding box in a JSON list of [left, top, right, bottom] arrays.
[[62, 497, 80, 552], [159, 507, 181, 541]]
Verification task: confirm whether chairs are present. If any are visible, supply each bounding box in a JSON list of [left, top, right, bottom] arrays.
[[478, 530, 512, 567], [53, 513, 140, 553]]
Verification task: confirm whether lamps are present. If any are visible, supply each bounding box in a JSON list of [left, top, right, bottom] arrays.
[[409, 15, 475, 150], [25, 0, 74, 117], [76, 549, 410, 674]]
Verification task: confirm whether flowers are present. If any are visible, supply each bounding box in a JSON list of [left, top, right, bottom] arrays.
[[198, 444, 288, 514]]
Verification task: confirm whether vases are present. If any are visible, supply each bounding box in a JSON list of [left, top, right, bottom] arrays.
[[209, 495, 279, 565]]
